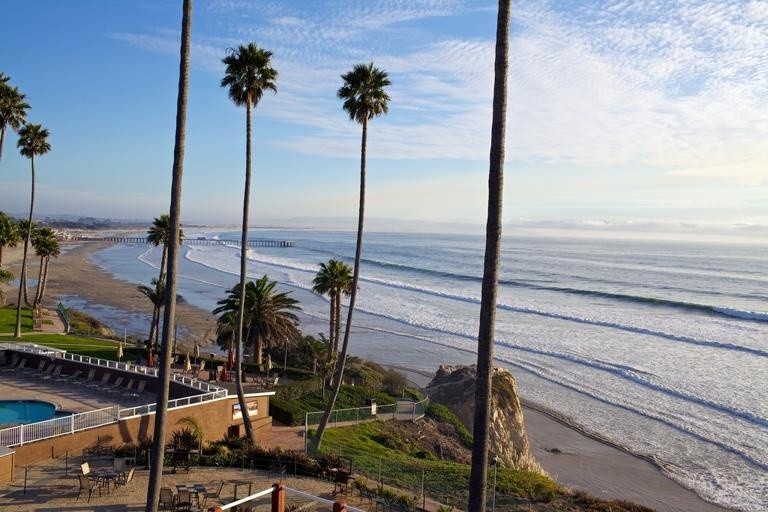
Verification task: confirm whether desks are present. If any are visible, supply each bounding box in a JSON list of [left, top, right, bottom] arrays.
[[220, 478, 252, 504]]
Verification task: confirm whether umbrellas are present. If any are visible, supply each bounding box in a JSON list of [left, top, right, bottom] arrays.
[[115, 341, 124, 361], [265, 354, 273, 381], [220, 362, 229, 383], [147, 346, 155, 367], [183, 353, 192, 372]]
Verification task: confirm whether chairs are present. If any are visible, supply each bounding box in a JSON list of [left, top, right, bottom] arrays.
[[0, 353, 280, 402], [77, 456, 224, 512]]
